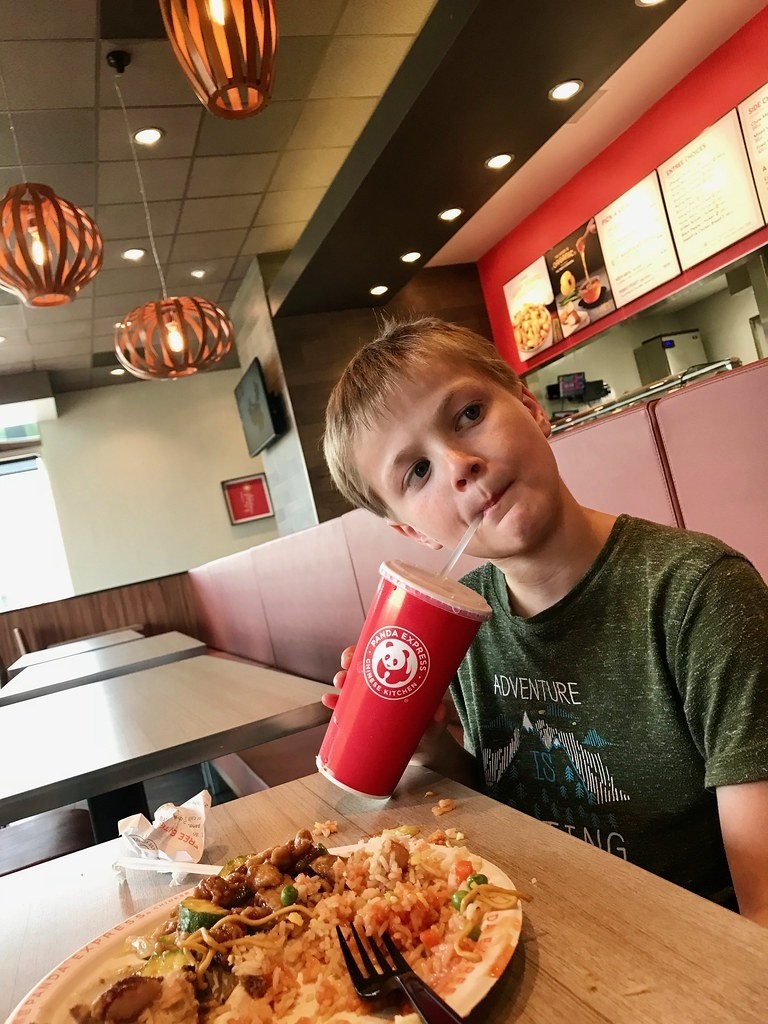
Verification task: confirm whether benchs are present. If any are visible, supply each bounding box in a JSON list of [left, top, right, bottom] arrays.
[[187, 360, 768, 799]]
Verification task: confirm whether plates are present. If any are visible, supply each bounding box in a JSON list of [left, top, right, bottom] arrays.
[[6, 844, 522, 1024], [512, 305, 551, 352]]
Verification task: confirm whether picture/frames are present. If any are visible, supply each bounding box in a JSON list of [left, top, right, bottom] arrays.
[[221, 472, 275, 527]]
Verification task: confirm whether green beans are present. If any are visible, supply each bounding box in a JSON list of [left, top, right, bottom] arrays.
[[466, 874, 488, 889], [281, 885, 298, 905], [453, 891, 469, 911]]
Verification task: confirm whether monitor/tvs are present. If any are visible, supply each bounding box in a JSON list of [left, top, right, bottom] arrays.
[[234, 357, 277, 458], [558, 372, 587, 398]]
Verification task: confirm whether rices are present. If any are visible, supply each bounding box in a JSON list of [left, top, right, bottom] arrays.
[[228, 832, 482, 1024]]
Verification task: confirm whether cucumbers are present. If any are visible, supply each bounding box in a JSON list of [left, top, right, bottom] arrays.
[[179, 899, 229, 932]]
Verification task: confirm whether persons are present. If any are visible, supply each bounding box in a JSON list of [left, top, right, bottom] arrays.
[[324, 318, 768, 929]]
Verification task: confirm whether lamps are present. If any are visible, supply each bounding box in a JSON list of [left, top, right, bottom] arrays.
[[107, 49, 234, 382], [0, 75, 103, 309], [158, 0, 280, 118]]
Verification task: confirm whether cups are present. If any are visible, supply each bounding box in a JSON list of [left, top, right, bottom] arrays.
[[580, 275, 601, 304], [317, 559, 492, 798]]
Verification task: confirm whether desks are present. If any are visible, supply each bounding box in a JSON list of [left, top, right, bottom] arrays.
[[0, 627, 768, 1024]]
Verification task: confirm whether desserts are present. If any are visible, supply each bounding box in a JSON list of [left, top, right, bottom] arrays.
[[557, 303, 587, 338]]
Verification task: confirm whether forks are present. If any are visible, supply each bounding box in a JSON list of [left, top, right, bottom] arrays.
[[336, 922, 466, 1024]]
